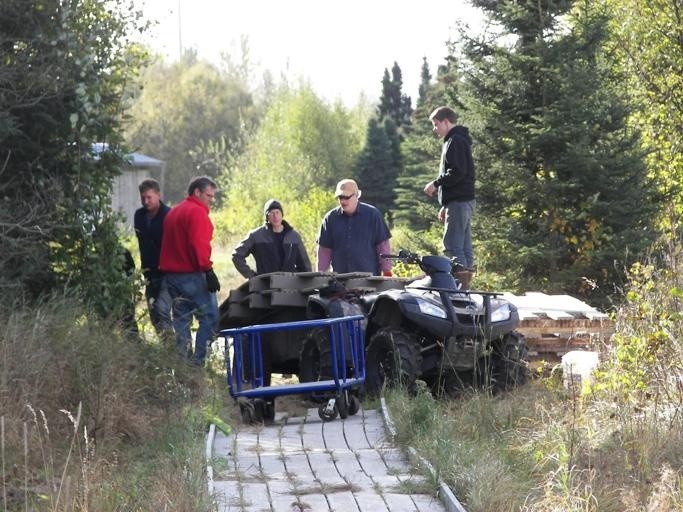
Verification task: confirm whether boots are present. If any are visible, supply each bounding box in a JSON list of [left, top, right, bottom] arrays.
[[454, 270, 473, 290]]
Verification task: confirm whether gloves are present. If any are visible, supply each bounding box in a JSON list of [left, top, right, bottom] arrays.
[[205, 268, 221, 292]]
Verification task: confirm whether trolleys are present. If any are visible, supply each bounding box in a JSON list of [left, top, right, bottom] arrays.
[[217, 313, 371, 426]]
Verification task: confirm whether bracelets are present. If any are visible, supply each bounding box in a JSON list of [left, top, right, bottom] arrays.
[[384, 272, 391, 276]]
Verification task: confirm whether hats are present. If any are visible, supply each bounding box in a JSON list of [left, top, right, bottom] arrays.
[[334, 179, 357, 199], [264, 199, 283, 218]]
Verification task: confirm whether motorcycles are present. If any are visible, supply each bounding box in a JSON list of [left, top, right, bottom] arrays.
[[289, 249, 529, 403]]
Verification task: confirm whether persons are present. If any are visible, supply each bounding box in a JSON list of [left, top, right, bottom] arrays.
[[231, 200, 313, 379], [158, 176, 220, 367], [423, 106, 477, 289], [133, 179, 177, 353], [119, 249, 139, 336], [317, 177, 392, 277]]
